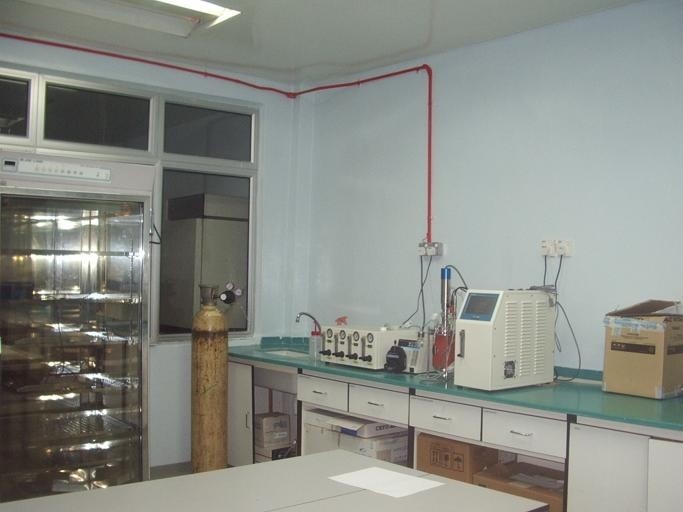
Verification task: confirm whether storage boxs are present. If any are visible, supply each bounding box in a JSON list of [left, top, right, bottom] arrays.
[[251, 410, 289, 448], [468, 459, 565, 512], [416, 431, 496, 482], [333, 427, 413, 470], [600, 299, 682, 400], [254, 439, 297, 463]]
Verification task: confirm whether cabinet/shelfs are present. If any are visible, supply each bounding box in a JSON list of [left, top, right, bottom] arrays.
[[561, 412, 682, 512], [407, 381, 569, 463], [159, 193, 250, 335], [296, 364, 412, 433], [0, 193, 149, 502], [222, 351, 300, 468]]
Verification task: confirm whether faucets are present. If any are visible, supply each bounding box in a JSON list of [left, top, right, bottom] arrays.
[[295, 311, 322, 333]]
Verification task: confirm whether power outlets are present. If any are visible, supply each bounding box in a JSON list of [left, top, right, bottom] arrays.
[[417, 241, 444, 257], [556, 240, 573, 257], [539, 239, 557, 257]]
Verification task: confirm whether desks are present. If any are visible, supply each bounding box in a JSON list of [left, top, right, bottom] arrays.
[[0, 443, 554, 512]]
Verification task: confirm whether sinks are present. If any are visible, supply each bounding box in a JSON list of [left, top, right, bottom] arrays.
[[252, 347, 310, 359]]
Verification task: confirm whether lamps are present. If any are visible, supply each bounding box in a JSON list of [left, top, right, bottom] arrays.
[[20, 0, 202, 40]]
